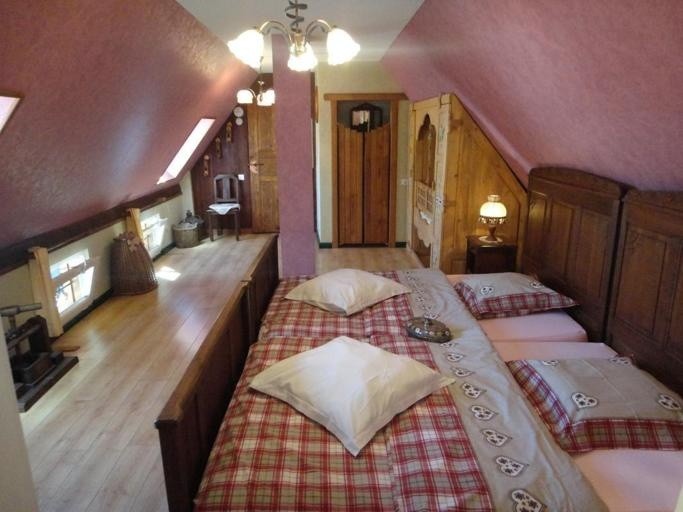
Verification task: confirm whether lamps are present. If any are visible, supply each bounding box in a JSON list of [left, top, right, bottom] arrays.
[[472, 193, 511, 247], [221, 0, 364, 106]]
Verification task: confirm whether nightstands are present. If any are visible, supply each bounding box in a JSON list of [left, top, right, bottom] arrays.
[[463, 233, 514, 272]]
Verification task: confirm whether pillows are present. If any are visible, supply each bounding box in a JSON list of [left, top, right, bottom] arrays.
[[252, 339, 453, 461], [507, 355, 678, 455], [284, 267, 412, 313], [455, 273, 576, 317]]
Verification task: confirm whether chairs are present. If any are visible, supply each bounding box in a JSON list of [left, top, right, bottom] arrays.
[[208, 172, 246, 239]]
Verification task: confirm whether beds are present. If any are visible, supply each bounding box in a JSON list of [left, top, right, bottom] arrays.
[[160, 191, 683, 512], [244, 165, 624, 342]]
